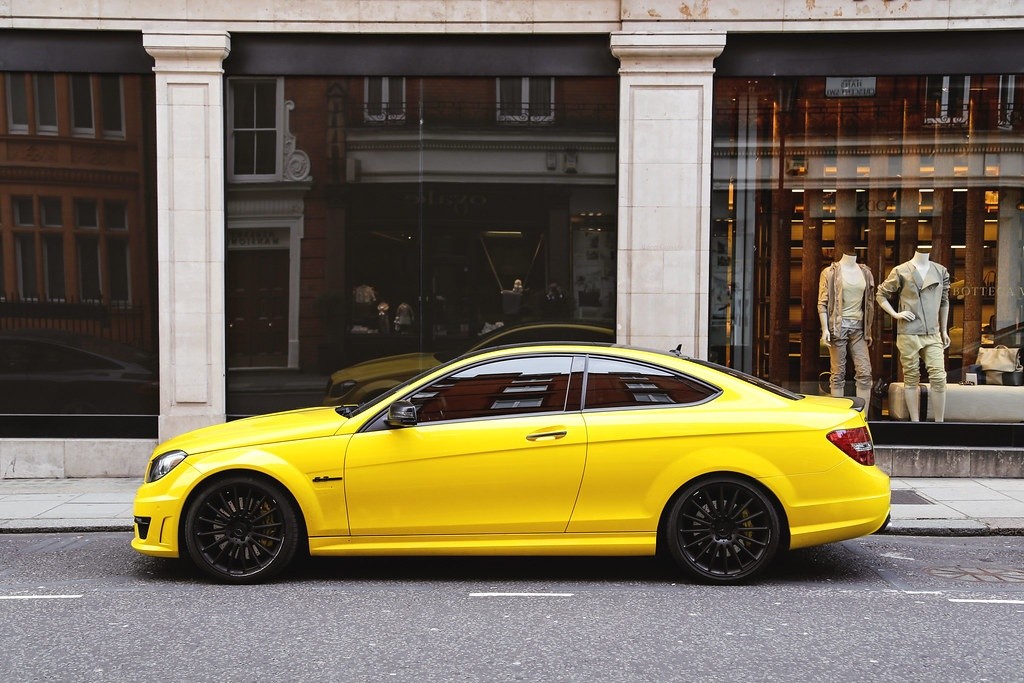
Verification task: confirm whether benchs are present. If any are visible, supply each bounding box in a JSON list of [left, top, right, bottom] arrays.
[[888, 382, 1024, 423]]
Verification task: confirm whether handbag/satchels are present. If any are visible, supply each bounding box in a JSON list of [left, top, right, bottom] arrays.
[[975, 344, 1024, 385]]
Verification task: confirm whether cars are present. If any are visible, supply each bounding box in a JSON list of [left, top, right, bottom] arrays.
[[871, 322, 1024, 423], [0, 329, 159, 438], [129, 342, 892, 584], [324, 321, 616, 407]]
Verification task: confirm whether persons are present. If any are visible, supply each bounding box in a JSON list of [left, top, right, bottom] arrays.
[[876, 250, 952, 422], [817, 253, 875, 422]]
